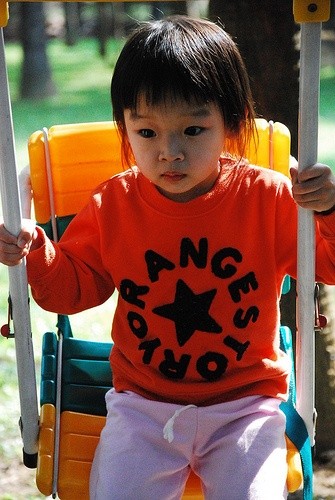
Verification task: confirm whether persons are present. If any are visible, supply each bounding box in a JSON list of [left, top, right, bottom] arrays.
[[0, 15, 335, 500]]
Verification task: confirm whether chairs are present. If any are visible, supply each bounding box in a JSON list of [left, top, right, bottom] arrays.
[[27, 117, 303, 500]]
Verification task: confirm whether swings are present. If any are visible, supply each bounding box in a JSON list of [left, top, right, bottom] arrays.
[[0, 0, 331, 500]]
